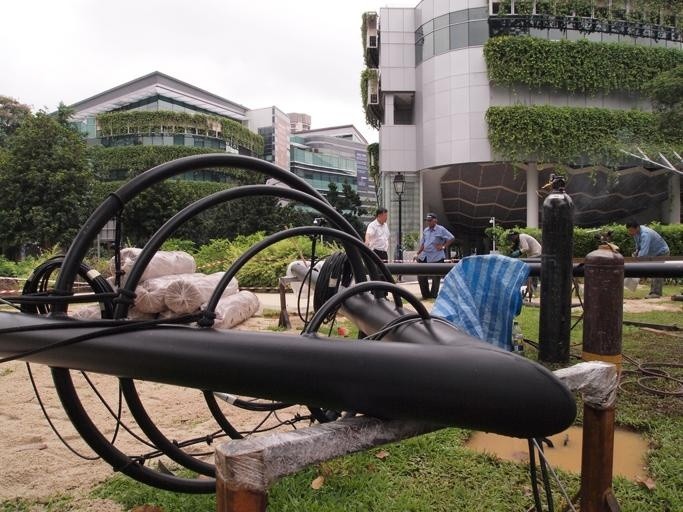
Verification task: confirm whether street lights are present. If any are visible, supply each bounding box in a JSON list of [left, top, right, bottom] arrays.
[[392, 171, 407, 262], [488, 216, 495, 252], [312, 218, 327, 248]]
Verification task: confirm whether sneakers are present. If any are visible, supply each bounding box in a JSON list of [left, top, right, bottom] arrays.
[[645, 292, 660, 299]]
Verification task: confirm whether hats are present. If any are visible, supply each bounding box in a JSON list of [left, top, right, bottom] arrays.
[[424, 213, 436, 220]]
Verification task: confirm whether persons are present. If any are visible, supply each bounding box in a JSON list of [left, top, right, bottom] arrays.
[[506, 228, 542, 294], [414, 212, 455, 300], [624, 215, 671, 301], [363, 206, 392, 300]]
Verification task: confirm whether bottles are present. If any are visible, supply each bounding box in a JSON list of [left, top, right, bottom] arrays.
[[510, 321, 524, 356]]
